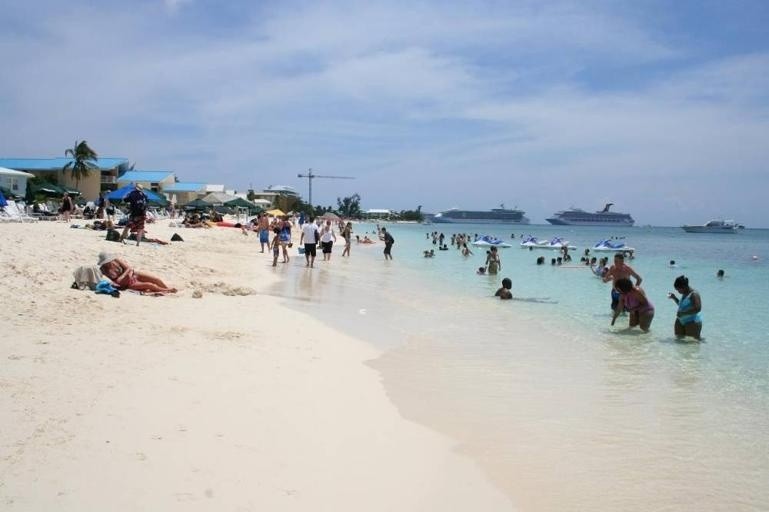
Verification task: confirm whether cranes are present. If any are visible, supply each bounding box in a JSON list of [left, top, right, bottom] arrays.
[[297, 168, 356, 209]]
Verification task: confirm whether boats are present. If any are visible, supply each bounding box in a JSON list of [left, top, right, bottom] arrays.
[[540, 241, 578, 252], [681, 216, 746, 234], [543, 201, 635, 227], [434, 204, 531, 225], [474, 236, 511, 249], [523, 237, 548, 250], [592, 240, 635, 254]]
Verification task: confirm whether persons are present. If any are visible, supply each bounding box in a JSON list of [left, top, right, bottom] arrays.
[[240, 209, 393, 267], [668, 275, 703, 338], [33, 183, 223, 246], [424, 231, 654, 332], [97, 249, 177, 294]]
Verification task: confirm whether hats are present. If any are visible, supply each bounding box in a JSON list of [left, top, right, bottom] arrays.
[[97, 251, 117, 265]]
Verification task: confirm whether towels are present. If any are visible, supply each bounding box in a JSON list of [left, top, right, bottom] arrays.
[[74, 263, 103, 290], [96, 279, 117, 295]]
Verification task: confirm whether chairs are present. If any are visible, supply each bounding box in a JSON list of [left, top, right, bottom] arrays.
[[0, 198, 186, 226]]
